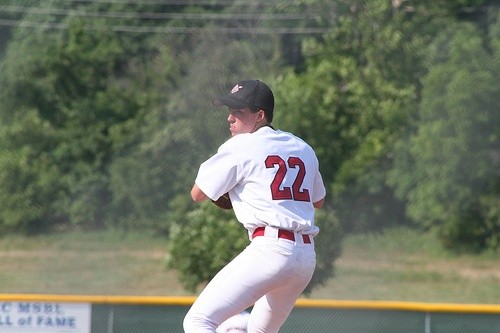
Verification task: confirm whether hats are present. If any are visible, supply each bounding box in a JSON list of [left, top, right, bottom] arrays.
[[213, 80, 274, 112]]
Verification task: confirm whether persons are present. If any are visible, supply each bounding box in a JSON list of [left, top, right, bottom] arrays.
[[183, 80, 325, 333]]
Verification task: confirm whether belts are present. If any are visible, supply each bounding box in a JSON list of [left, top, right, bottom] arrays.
[[251, 227, 311, 243]]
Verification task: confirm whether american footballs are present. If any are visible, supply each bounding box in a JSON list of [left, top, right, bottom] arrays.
[[211, 193, 233, 209]]
